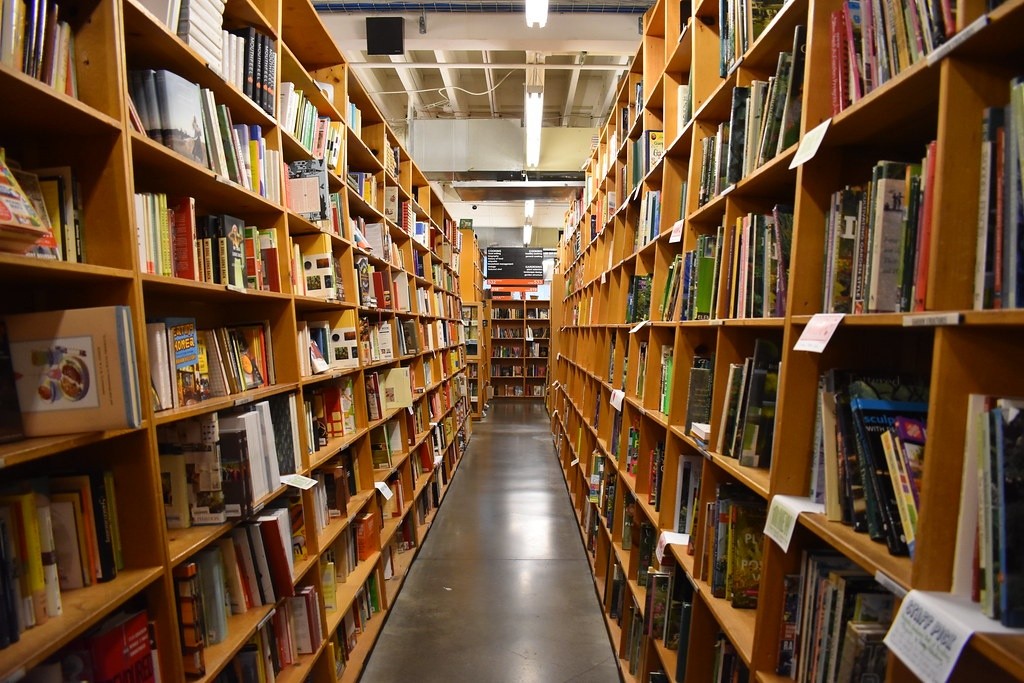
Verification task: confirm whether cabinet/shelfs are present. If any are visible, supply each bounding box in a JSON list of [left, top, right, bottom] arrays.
[[0, 0, 1024, 683]]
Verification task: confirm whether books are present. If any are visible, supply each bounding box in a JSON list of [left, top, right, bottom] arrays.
[[557, 1, 1023, 682], [0, 2, 478, 682], [482, 307, 549, 396]]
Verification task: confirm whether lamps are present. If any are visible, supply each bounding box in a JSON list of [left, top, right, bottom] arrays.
[[526, 84, 543, 167]]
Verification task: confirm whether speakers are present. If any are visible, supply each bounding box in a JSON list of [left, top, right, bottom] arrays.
[[365, 17, 405, 56]]
